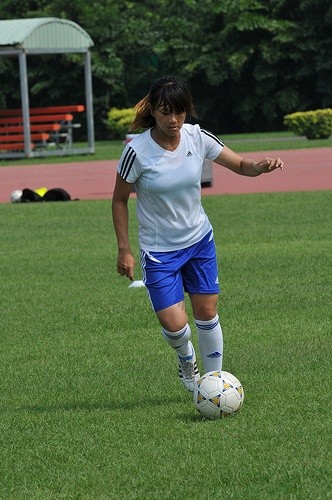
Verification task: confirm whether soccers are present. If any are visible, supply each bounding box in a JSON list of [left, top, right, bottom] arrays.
[[193, 370, 245, 420], [9, 189, 23, 203]]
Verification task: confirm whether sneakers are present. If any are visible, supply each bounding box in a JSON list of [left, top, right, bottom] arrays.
[[177, 340, 200, 392]]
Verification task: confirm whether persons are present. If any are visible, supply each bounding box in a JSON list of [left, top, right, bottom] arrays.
[[111, 75, 285, 394]]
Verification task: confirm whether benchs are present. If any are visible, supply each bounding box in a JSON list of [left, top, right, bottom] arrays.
[[0, 105, 84, 153]]
